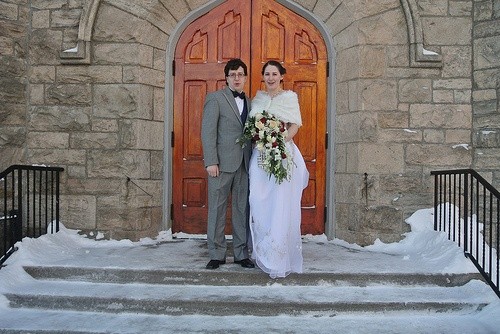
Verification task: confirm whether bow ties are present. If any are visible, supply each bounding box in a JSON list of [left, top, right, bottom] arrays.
[[229, 88, 244, 99]]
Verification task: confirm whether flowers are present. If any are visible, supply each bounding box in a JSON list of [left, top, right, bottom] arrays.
[[236, 110, 297, 185]]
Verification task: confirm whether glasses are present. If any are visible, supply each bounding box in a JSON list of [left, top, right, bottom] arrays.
[[228, 72, 245, 78]]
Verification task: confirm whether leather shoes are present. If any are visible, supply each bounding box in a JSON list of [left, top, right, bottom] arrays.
[[206, 260, 226, 269], [234, 258, 255, 268]]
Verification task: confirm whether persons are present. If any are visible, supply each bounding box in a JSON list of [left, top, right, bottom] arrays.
[[242, 59, 310, 279], [201, 58, 255, 270]]
